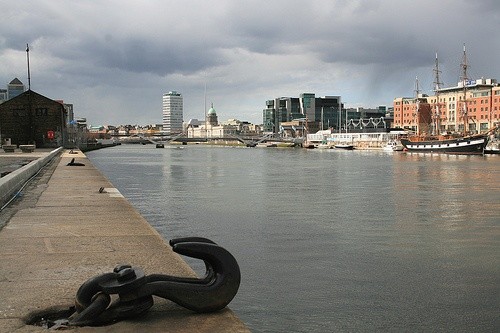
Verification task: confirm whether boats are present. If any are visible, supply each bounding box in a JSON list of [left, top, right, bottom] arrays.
[[484, 145, 500, 156], [334, 144, 354, 150], [391, 141, 405, 151], [156, 143, 164, 148]]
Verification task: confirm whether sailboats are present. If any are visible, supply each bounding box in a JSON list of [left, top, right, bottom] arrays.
[[400, 42, 497, 158]]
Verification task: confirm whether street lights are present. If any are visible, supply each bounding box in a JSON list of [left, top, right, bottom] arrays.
[[24, 42, 36, 145]]
